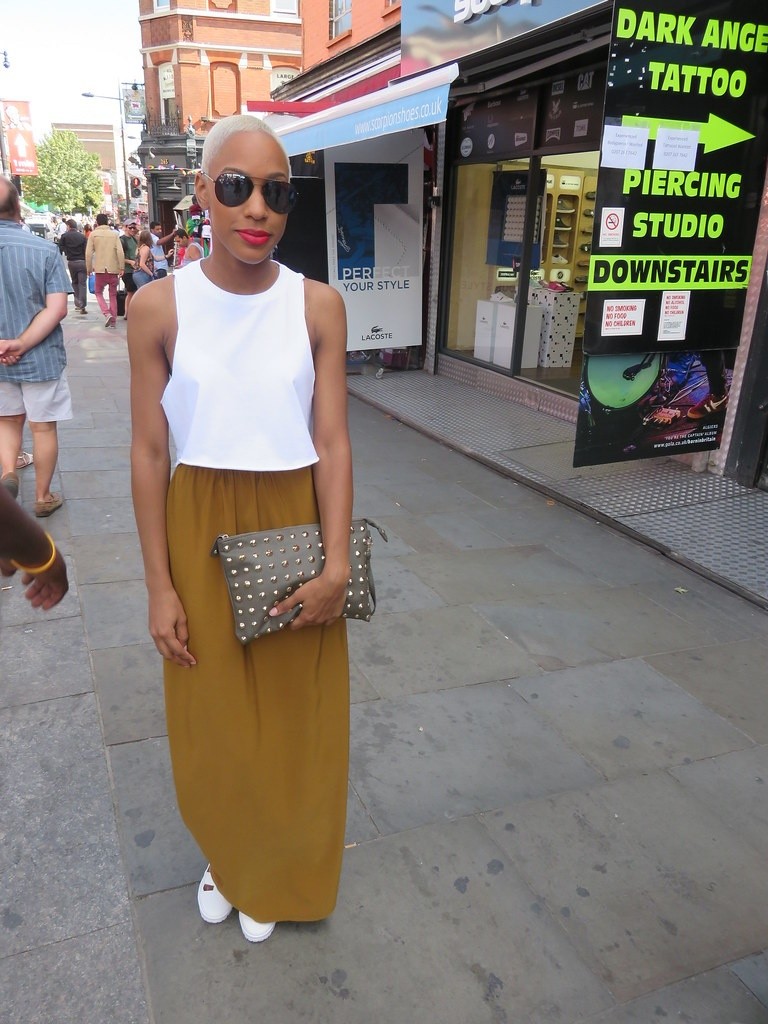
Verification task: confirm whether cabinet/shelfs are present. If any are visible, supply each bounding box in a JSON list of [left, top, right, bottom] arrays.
[[473, 300, 543, 369], [489, 161, 598, 338]]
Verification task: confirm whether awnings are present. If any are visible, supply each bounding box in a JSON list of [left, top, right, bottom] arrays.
[[261, 64, 460, 159]]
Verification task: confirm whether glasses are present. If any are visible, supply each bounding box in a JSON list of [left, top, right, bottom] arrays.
[[127, 226, 137, 229], [204, 172, 302, 214]]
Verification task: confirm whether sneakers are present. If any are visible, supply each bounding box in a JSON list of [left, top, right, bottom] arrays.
[[526, 192, 598, 292], [490, 292, 513, 302], [688, 392, 730, 419]]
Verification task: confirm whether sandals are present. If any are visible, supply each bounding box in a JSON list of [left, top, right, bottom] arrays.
[[16, 451, 33, 469]]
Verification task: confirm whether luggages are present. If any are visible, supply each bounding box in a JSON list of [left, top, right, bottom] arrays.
[[117, 276, 127, 316]]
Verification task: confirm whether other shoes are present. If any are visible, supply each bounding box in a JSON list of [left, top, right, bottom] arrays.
[[111, 325, 114, 327], [239, 910, 276, 943], [1, 472, 19, 499], [197, 862, 235, 923], [76, 307, 87, 313], [105, 314, 113, 327], [35, 493, 63, 517]]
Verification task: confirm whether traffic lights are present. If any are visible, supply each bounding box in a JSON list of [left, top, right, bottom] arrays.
[[130, 176, 142, 198]]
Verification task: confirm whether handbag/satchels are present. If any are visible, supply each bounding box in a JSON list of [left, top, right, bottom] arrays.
[[209, 516, 389, 645], [89, 272, 96, 293]]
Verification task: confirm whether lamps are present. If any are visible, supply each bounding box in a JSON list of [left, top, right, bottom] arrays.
[[148, 146, 156, 159], [166, 179, 182, 189]]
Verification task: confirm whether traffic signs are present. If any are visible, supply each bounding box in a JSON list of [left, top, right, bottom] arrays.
[[580, 1, 768, 355]]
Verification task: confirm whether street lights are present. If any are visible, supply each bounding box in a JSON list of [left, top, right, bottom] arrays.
[[81, 81, 129, 219]]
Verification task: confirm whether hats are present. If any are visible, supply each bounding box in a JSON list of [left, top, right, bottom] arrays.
[[124, 219, 138, 227]]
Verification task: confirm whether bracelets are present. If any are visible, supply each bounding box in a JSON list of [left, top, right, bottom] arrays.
[[10, 533, 56, 573]]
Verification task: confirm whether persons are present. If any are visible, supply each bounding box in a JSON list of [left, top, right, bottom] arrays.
[[52, 213, 205, 328], [126, 116, 351, 942], [0, 485, 69, 611], [0, 173, 75, 517]]
